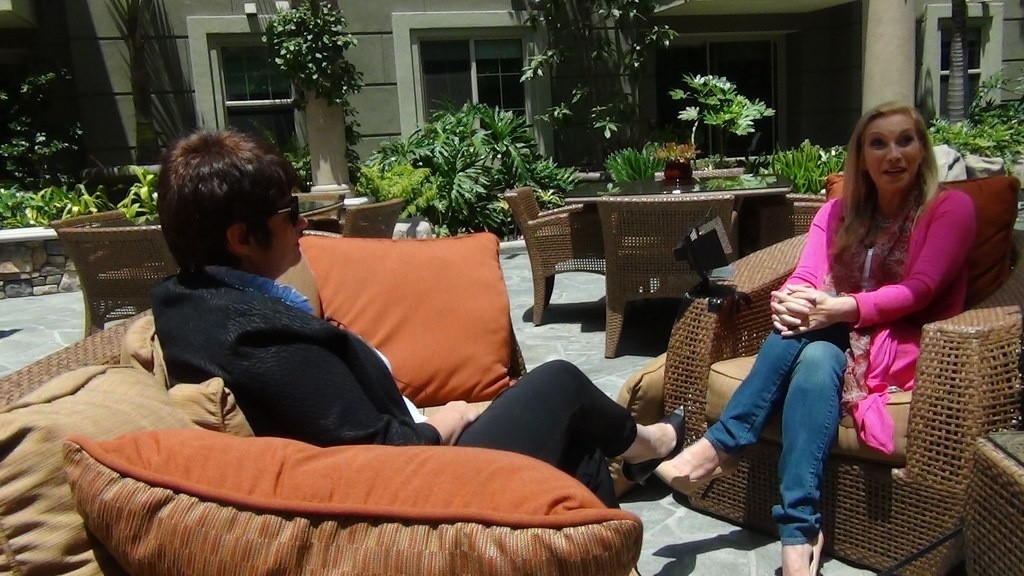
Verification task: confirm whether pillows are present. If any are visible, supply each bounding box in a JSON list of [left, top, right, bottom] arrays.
[[825, 172, 1021, 307], [606, 351, 667, 496], [0, 316, 254, 576], [61, 426, 645, 576], [300, 230, 522, 405]]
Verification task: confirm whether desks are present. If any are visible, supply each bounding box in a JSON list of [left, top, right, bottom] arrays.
[[563, 174, 793, 204]]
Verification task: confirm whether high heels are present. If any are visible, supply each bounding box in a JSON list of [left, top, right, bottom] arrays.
[[809, 528, 825, 576], [655, 446, 740, 504], [622, 404, 686, 486]]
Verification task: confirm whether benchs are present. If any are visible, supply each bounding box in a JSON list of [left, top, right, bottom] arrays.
[[0, 304, 156, 406]]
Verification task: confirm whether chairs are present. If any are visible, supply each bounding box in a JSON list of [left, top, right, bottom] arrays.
[[505, 185, 1024, 576], [323, 196, 407, 239], [51, 211, 178, 341]]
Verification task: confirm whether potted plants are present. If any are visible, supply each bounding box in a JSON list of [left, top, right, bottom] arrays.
[[657, 142, 702, 187], [604, 141, 669, 194], [356, 164, 444, 241]]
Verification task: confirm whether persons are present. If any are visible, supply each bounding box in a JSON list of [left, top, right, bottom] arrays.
[[151, 129, 685, 508], [653, 100, 976, 576]]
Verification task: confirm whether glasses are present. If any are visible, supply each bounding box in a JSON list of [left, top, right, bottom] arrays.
[[272, 196, 300, 227]]
